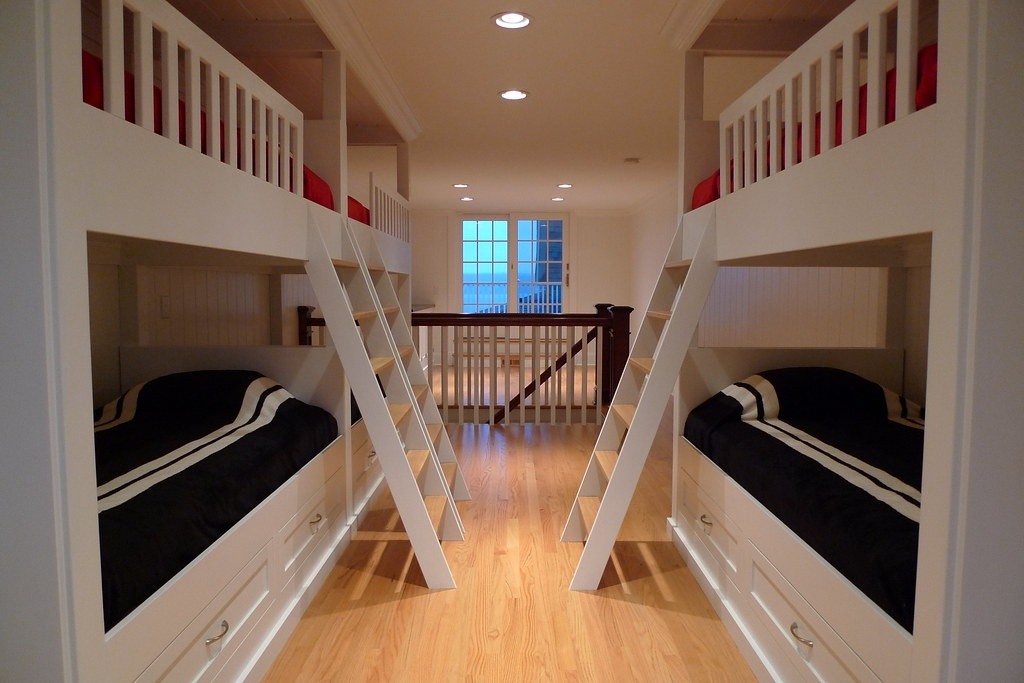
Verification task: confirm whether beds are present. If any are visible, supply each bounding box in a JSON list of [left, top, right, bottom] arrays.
[[663, 0, 1024, 683], [0, 0, 434, 683]]
